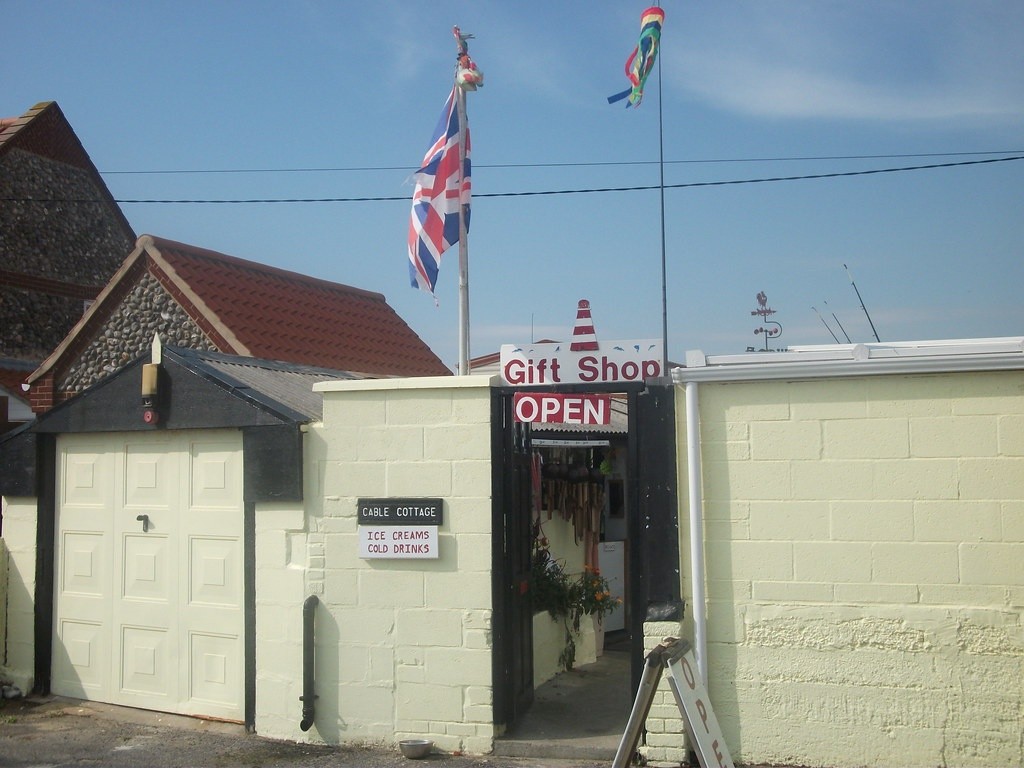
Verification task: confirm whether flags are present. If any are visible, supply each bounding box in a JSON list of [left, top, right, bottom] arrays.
[[409, 88, 472, 294]]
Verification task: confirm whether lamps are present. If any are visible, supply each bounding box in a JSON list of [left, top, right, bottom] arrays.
[[141, 362, 158, 408]]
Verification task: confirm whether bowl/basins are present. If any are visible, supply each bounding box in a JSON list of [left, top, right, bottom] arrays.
[[400, 740, 433, 760]]
